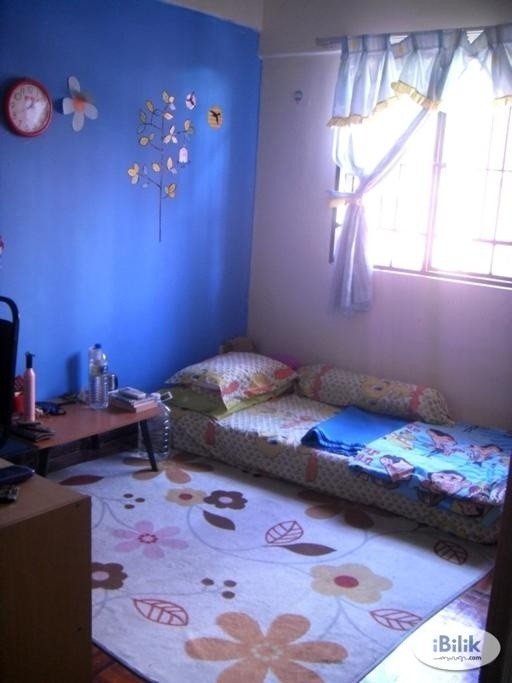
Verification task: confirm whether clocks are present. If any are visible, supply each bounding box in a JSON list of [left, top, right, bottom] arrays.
[[7, 80, 54, 138]]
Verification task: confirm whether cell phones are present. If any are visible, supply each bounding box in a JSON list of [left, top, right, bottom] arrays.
[[0, 464, 37, 488]]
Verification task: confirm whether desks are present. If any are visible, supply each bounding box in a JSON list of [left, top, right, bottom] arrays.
[[5, 397, 160, 477]]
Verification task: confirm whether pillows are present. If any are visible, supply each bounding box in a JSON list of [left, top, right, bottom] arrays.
[[155, 352, 302, 421], [294, 364, 457, 427]]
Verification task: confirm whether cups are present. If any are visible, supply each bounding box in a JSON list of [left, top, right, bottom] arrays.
[[14, 392, 24, 415], [108, 374, 119, 390]]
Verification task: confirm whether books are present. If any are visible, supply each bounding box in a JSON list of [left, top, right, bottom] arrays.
[[108, 387, 158, 413]]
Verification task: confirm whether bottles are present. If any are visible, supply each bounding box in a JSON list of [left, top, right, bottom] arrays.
[[88, 343, 109, 409]]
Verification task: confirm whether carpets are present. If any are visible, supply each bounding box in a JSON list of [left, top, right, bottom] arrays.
[[44, 445, 497, 682]]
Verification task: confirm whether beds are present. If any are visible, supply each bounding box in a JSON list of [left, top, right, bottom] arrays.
[[140, 387, 512, 545]]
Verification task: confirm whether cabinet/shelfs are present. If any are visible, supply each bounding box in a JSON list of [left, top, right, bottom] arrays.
[[0, 456, 93, 682]]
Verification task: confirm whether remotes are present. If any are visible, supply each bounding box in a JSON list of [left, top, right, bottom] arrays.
[[120, 387, 146, 398]]
